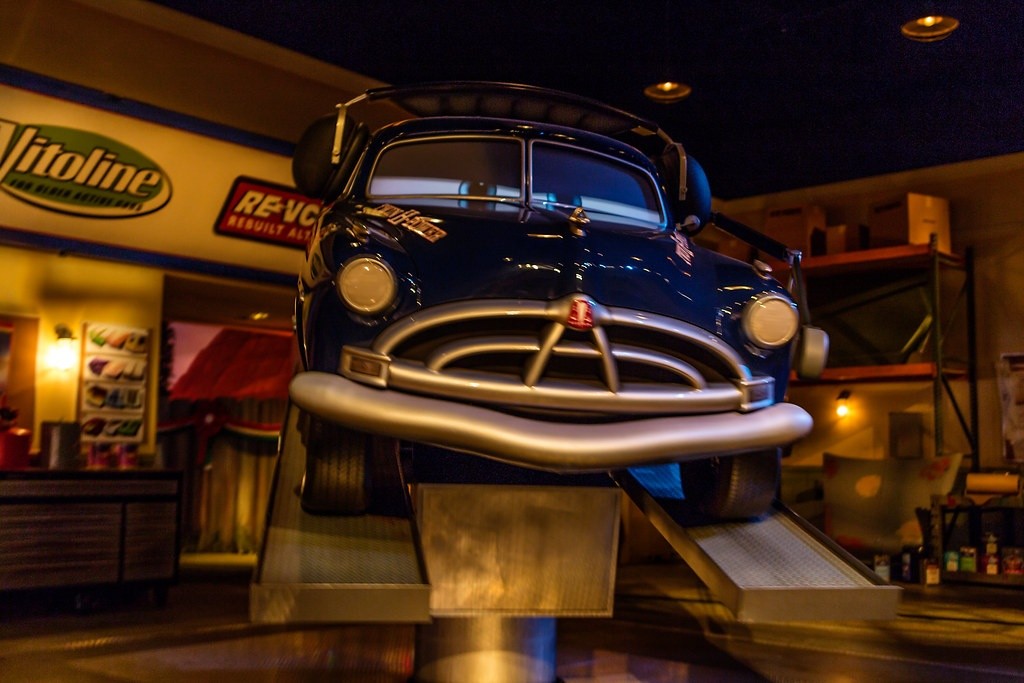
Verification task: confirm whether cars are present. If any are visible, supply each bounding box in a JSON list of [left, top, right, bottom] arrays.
[[283, 113, 814, 527]]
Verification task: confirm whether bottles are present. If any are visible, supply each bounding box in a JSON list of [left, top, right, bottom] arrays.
[[871, 524, 1022, 591]]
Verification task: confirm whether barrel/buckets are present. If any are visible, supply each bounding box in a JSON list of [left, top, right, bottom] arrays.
[[39, 420, 81, 470]]
